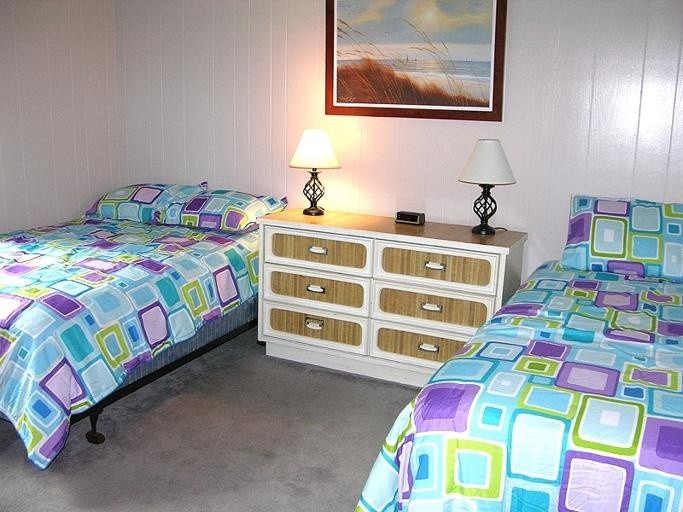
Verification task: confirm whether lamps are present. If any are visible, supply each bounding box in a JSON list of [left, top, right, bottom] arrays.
[[456, 135, 517, 238], [287, 128, 343, 216]]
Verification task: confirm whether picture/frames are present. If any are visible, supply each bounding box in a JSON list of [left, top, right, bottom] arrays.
[[323, 0, 509, 123]]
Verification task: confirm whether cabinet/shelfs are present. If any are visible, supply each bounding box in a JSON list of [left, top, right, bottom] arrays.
[[257, 207, 528, 389]]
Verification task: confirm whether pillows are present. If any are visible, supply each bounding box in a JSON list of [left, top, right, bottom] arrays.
[[558, 190, 683, 279], [80, 179, 209, 223], [147, 188, 290, 236]]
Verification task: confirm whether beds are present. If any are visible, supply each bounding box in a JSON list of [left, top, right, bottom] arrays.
[[353, 258, 682, 511], [0, 219, 258, 472]]
